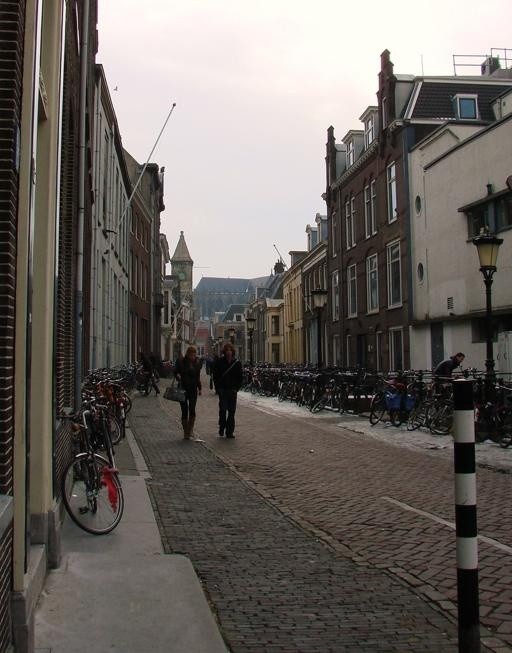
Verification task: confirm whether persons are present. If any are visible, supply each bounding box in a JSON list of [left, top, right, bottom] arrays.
[[433, 352, 465, 394], [136, 343, 243, 440]]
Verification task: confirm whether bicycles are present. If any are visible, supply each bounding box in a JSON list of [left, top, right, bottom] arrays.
[[238, 358, 512, 449], [57, 361, 163, 535]]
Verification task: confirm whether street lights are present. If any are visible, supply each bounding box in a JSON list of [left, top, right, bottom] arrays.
[[469, 223, 504, 400], [228, 325, 235, 343], [211, 336, 224, 357], [245, 314, 256, 365], [310, 283, 328, 369]]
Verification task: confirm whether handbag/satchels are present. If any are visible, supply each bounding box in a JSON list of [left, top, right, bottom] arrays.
[[163, 374, 186, 402]]
